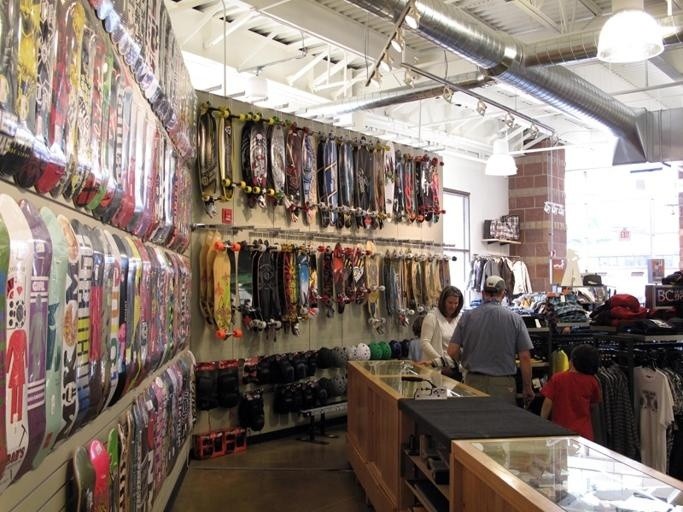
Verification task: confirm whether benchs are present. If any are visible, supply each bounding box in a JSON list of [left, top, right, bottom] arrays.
[[295, 402, 347, 446]]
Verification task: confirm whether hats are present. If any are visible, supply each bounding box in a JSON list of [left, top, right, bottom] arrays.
[[484, 276, 508, 293], [571, 344, 598, 374]]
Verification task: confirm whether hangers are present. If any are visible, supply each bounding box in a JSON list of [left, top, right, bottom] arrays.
[[529, 334, 546, 349], [595, 346, 677, 371], [554, 334, 609, 352]]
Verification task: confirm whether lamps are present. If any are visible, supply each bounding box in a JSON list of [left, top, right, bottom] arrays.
[[482, 128, 518, 177], [400, 64, 555, 139], [365, 0, 421, 87], [596, 0, 664, 65]]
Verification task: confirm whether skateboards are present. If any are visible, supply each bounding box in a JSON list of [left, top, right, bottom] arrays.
[[2, 0, 197, 508], [195, 100, 446, 229], [199, 230, 456, 340]]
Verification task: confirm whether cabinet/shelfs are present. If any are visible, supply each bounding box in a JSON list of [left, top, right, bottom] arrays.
[[343, 362, 559, 512]]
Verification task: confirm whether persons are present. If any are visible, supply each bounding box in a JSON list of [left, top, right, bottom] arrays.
[[540, 344, 602, 442], [408, 275, 535, 406]]
